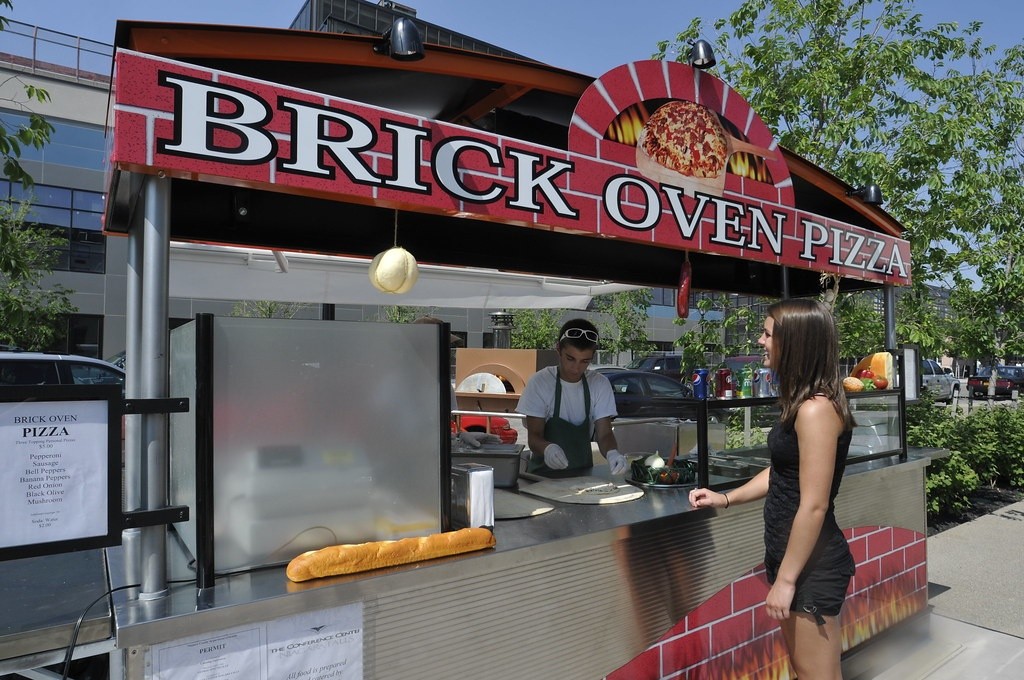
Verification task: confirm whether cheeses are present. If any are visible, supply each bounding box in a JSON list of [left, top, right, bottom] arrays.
[[851, 352, 892, 390]]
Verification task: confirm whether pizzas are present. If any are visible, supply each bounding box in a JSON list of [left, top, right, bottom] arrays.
[[640, 100, 727, 178]]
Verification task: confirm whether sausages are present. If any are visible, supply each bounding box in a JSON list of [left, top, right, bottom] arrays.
[[677, 262, 691, 317]]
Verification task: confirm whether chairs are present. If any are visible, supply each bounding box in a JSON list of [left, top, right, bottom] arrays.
[[626, 382, 641, 393]]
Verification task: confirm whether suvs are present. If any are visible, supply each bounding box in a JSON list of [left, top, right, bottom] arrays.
[[592, 351, 699, 396]]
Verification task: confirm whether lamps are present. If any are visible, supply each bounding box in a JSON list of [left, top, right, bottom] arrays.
[[371, 17, 428, 60], [367, 208, 419, 295], [688, 38, 717, 69]]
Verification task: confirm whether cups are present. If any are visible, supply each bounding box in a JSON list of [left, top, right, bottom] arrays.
[[624, 451, 654, 471]]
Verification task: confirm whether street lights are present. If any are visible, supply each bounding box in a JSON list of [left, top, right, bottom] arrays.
[[698, 305, 708, 335]]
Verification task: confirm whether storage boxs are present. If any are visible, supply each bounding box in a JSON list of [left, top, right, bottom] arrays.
[[453, 443, 526, 489], [451, 463, 495, 530]]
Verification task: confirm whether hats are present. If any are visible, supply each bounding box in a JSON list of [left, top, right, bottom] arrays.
[[411, 314, 462, 343]]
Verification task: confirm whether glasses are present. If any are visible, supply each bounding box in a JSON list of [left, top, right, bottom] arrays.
[[559, 328, 598, 351]]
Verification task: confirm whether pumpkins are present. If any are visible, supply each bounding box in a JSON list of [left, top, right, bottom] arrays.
[[843, 377, 864, 391]]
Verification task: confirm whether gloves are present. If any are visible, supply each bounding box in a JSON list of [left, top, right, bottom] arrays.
[[458, 431, 503, 447], [543, 443, 568, 469], [606, 449, 628, 475]]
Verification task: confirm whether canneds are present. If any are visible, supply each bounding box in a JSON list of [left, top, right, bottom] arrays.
[[771, 370, 781, 396], [736, 369, 753, 398], [715, 369, 732, 399], [692, 369, 710, 399], [754, 368, 770, 397]]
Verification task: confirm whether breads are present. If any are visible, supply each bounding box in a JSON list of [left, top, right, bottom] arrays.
[[286, 527, 496, 582]]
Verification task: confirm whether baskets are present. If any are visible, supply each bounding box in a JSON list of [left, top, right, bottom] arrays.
[[631, 457, 697, 484]]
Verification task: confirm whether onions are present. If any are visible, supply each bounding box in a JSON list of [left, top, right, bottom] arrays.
[[644, 450, 665, 469]]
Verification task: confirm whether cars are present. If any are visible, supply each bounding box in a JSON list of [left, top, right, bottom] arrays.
[[921, 358, 961, 409], [970, 365, 1024, 400], [715, 355, 765, 398], [601, 369, 724, 425]]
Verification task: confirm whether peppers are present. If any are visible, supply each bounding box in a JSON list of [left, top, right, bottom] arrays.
[[861, 378, 876, 390]]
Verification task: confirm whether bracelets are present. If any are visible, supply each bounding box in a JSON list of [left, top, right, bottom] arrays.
[[724, 494, 729, 509]]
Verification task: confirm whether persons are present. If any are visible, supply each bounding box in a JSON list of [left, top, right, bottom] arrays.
[[411, 317, 503, 447], [689, 296, 855, 680], [515, 319, 627, 475]]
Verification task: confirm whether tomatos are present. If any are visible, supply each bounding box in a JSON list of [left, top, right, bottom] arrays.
[[873, 375, 888, 389]]
